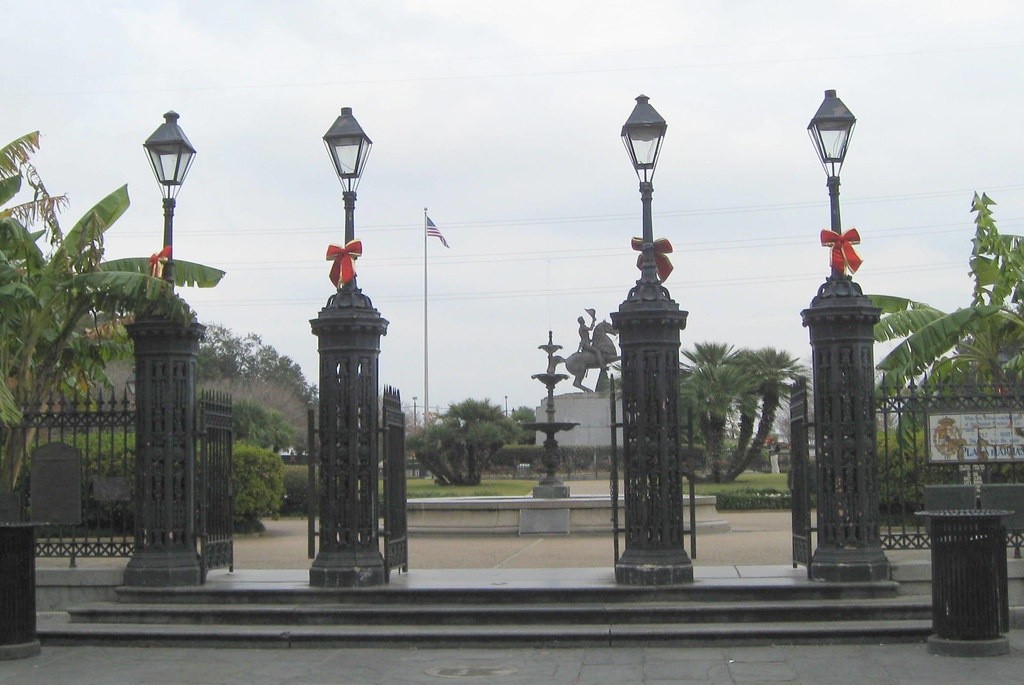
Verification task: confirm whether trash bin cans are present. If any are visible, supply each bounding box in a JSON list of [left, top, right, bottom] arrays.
[[0, 520, 53, 644], [915, 508, 1017, 643]]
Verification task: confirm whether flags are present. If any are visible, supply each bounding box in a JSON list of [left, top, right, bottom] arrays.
[[426, 216, 450, 249]]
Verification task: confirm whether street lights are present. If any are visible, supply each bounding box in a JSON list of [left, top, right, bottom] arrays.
[[303, 103, 393, 588], [605, 93, 696, 584], [413, 396, 424, 432], [808, 89, 893, 582], [505, 395, 511, 419], [118, 109, 246, 596]]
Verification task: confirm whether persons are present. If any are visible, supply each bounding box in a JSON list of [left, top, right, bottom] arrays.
[[767, 436, 781, 474], [577, 315, 605, 368]]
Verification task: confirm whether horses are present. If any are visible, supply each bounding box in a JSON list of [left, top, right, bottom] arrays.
[[547, 318, 623, 395]]
